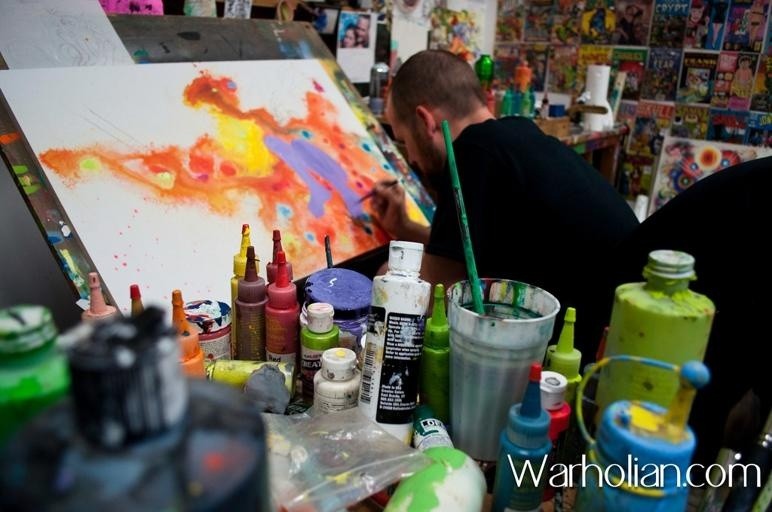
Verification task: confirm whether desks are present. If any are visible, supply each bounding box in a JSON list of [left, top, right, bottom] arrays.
[[559, 123, 629, 188]]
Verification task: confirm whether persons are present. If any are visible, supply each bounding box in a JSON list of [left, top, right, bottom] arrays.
[[340, 14, 371, 49], [369, 48, 648, 382], [343, 23, 357, 48], [637, 154, 770, 470]]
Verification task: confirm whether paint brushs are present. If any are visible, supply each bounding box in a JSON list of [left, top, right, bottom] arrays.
[[355, 179, 398, 205], [441, 120, 486, 317]]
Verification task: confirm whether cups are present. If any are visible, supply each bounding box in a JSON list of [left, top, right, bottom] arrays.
[[447, 279, 560, 463]]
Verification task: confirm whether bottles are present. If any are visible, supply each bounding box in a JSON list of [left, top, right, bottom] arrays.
[[82, 271, 205, 380], [229, 223, 450, 446], [492, 250, 719, 500], [474, 54, 550, 119]]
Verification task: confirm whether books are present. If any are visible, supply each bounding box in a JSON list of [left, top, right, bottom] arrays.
[[492, 0, 772, 223]]
